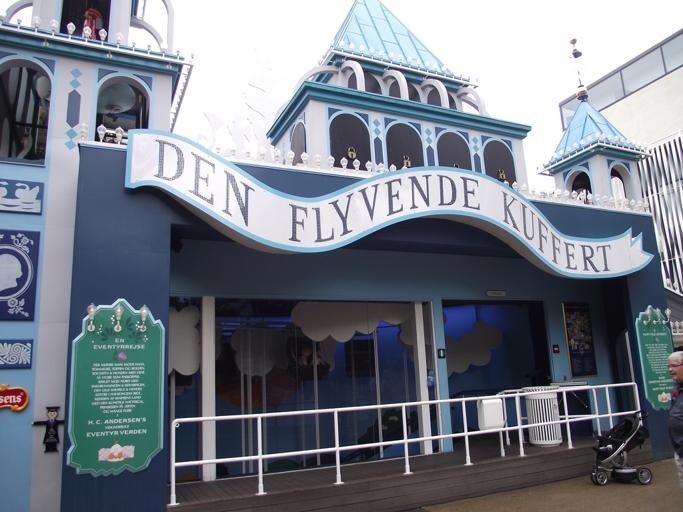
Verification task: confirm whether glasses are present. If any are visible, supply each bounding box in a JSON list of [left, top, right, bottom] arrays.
[[667, 364, 683, 369]]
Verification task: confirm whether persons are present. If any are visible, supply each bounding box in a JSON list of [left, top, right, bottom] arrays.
[[664, 350, 683, 466]]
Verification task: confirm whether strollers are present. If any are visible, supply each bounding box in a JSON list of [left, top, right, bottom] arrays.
[[590, 410, 655, 488]]
